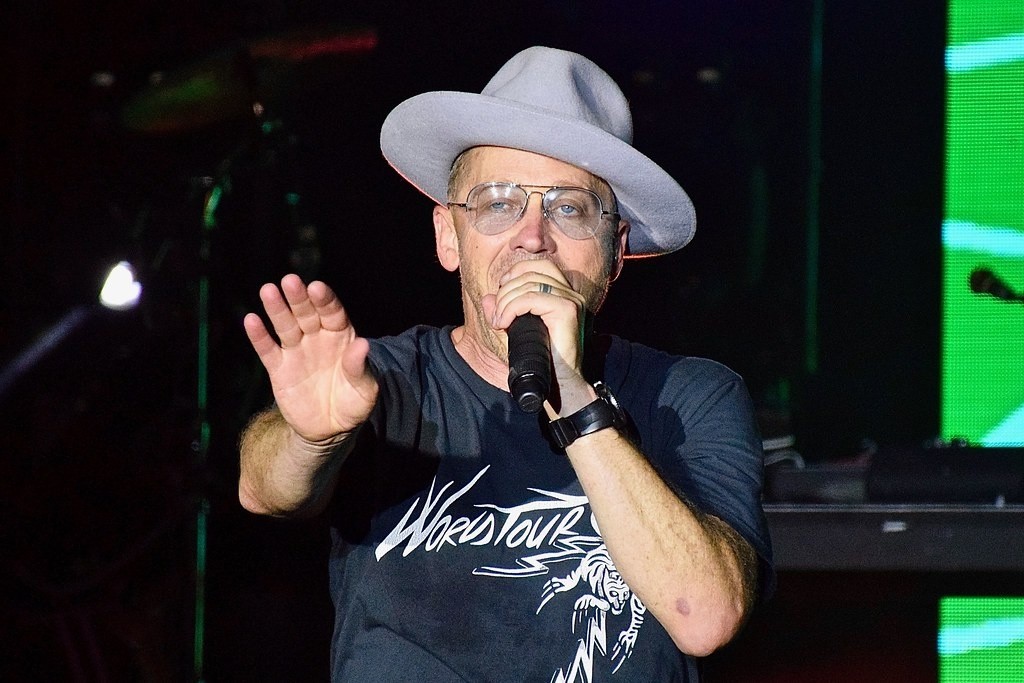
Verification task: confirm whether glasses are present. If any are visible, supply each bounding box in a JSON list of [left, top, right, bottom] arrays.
[[447, 182, 620, 241]]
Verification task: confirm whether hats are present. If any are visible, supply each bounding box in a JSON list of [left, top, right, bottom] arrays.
[[379, 46, 696, 258]]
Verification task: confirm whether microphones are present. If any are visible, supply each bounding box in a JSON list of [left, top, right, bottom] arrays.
[[970, 270, 1017, 302], [505, 312, 554, 413]]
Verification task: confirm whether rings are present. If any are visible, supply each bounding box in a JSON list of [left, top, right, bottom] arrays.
[[539, 282, 551, 294]]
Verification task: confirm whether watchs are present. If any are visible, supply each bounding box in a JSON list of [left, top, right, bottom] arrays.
[[541, 380, 629, 455]]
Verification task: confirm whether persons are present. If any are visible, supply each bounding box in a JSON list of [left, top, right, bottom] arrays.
[[238, 45, 768, 683]]
